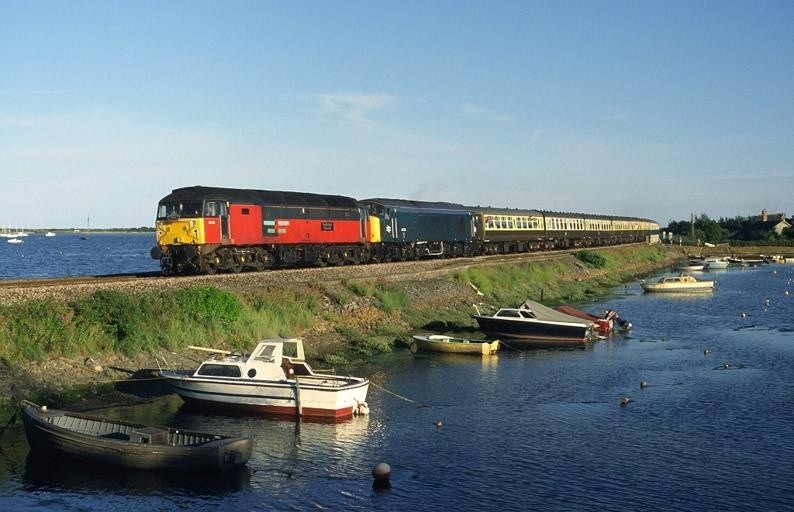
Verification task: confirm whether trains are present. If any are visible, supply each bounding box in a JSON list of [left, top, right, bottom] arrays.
[[149, 185, 662, 275]]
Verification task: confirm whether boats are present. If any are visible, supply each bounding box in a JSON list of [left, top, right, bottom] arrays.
[[411, 334, 500, 356], [0, 233, 30, 244], [689, 255, 730, 269], [157, 337, 370, 417], [472, 298, 594, 347], [18, 398, 253, 470], [674, 265, 705, 271], [727, 257, 764, 265], [553, 304, 632, 333], [639, 272, 717, 293], [44, 231, 56, 237]]
